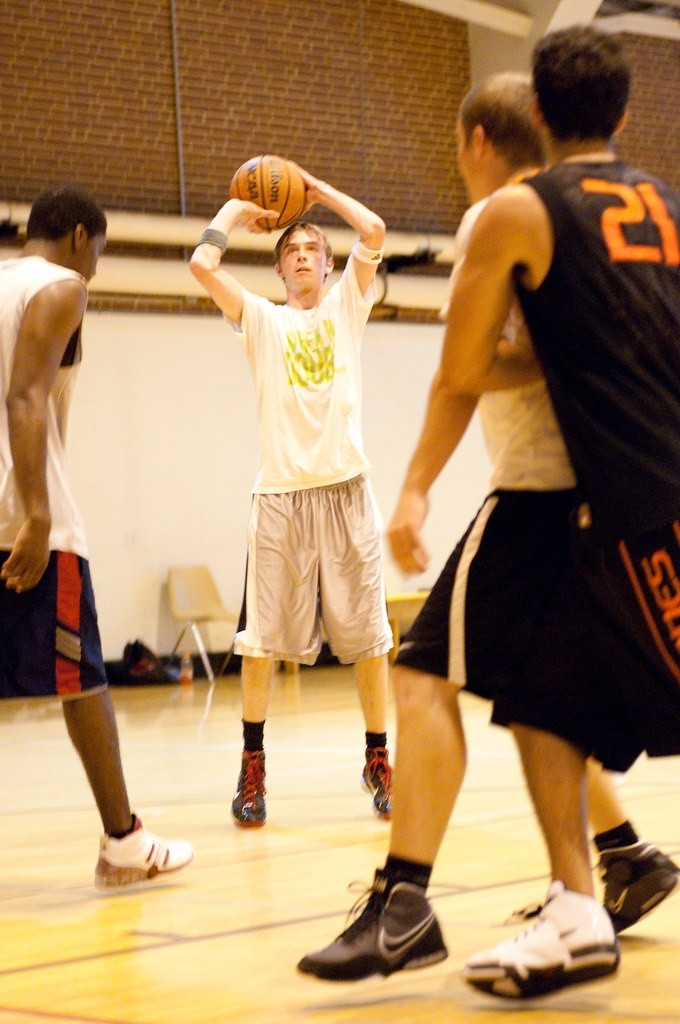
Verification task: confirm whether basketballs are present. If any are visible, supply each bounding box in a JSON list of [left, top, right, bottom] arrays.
[[232, 154, 306, 231]]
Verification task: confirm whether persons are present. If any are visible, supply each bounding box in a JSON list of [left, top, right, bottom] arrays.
[[296, 73, 680, 984], [188, 156, 387, 828], [438, 20, 678, 999], [1, 187, 195, 889]]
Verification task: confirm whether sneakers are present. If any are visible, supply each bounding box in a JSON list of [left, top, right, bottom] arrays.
[[295, 867, 451, 984], [600, 841, 680, 936], [231, 750, 268, 827], [362, 747, 393, 820], [462, 877, 625, 1000], [92, 813, 196, 891]]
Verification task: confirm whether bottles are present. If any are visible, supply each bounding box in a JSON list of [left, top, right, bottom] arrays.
[[178, 654, 193, 686]]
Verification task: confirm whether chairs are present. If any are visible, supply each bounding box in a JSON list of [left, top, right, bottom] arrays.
[[167, 565, 244, 680]]
